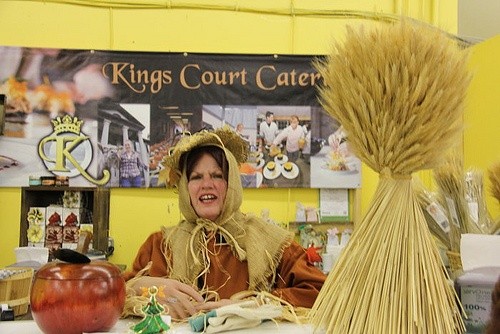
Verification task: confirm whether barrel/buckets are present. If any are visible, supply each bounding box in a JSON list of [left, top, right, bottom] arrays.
[[0, 267, 34, 318]]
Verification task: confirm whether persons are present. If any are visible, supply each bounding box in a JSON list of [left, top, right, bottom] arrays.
[[122, 126, 326, 319], [257, 111, 280, 159], [237, 123, 245, 138], [273, 116, 305, 162], [121, 138, 148, 188]]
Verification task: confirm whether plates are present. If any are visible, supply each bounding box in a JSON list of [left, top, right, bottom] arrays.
[[255, 153, 300, 179], [318, 151, 356, 175]]
